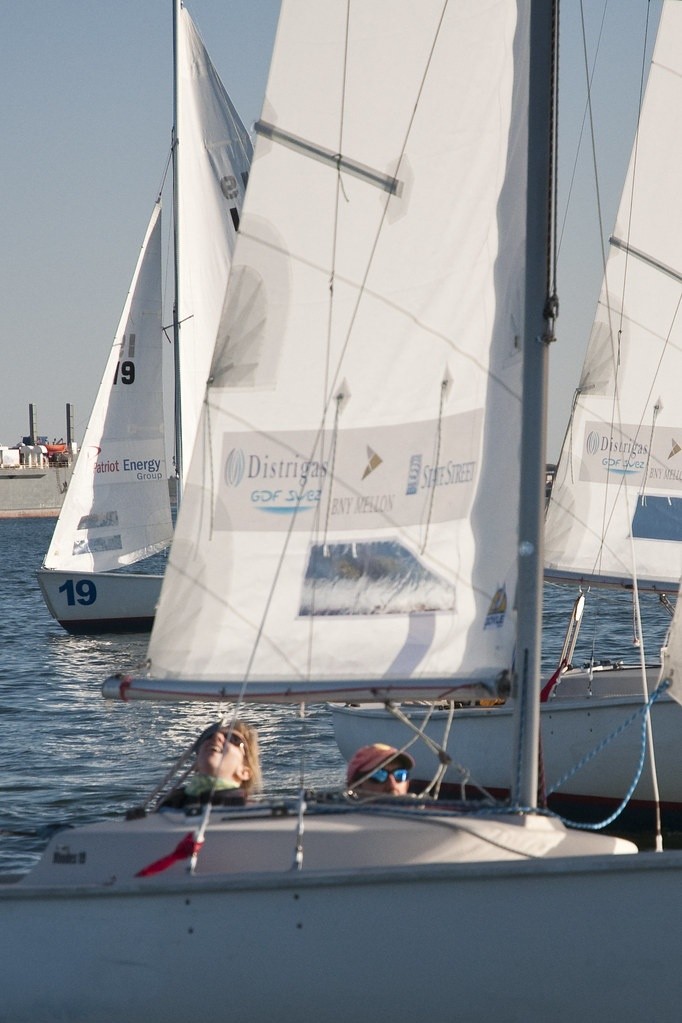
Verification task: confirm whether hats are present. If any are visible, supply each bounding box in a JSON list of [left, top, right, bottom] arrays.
[[347, 742, 413, 785]]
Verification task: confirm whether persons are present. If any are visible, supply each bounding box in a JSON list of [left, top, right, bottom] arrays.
[[348, 742, 415, 795], [158, 719, 263, 808]]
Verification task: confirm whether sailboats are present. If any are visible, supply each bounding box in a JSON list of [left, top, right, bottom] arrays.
[[0, 0, 675, 1019]]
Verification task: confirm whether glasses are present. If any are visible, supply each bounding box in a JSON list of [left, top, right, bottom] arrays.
[[208, 732, 246, 753], [364, 767, 410, 783]]
